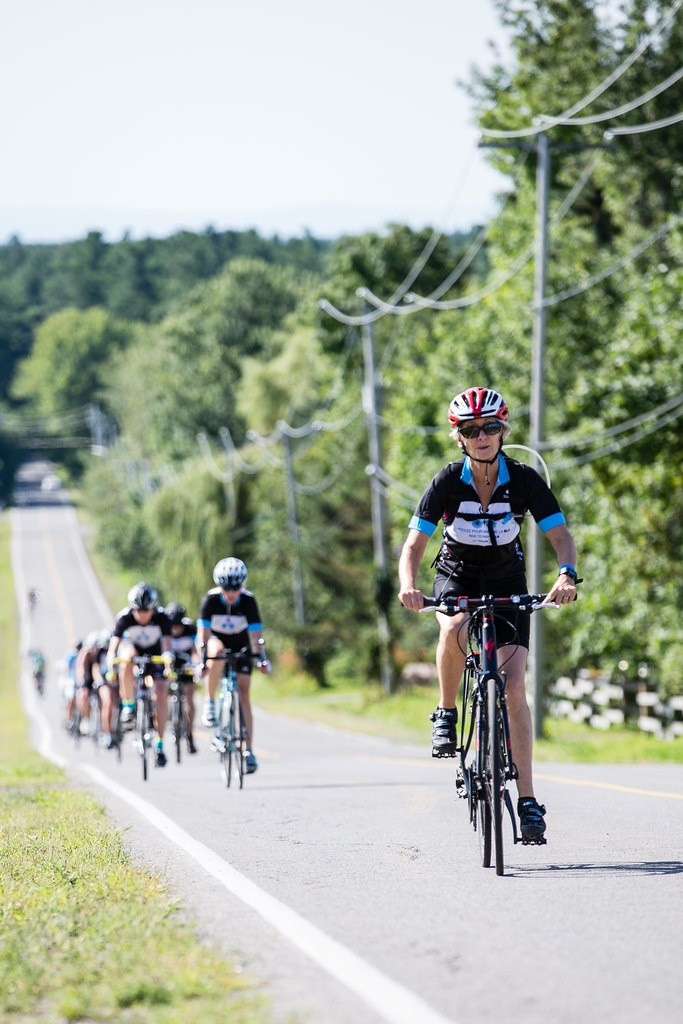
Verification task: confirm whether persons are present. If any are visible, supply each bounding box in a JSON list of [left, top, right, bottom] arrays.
[[21, 556, 267, 775], [397, 390, 576, 836]]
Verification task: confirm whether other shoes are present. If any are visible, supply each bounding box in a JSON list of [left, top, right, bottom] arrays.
[[245, 747, 257, 771], [120, 700, 135, 722], [186, 734, 196, 753], [155, 748, 166, 766], [200, 701, 215, 726]]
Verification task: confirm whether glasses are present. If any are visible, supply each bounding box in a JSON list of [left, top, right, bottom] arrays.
[[457, 421, 503, 439], [221, 584, 241, 591]]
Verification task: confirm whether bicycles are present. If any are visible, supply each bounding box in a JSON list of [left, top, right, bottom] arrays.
[[103, 654, 176, 780], [168, 657, 191, 762], [203, 648, 268, 790], [399, 591, 579, 877]]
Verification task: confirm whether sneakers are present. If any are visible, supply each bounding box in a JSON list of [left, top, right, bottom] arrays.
[[518, 797, 546, 835], [429, 705, 459, 753]]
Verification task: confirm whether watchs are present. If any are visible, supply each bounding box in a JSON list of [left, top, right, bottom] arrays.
[[557, 567, 583, 585]]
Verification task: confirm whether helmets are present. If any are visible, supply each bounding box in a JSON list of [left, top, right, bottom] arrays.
[[212, 557, 247, 585], [128, 582, 159, 610], [448, 387, 510, 428], [165, 601, 185, 619]]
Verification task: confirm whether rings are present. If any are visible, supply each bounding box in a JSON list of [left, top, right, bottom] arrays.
[[563, 597, 569, 599]]
